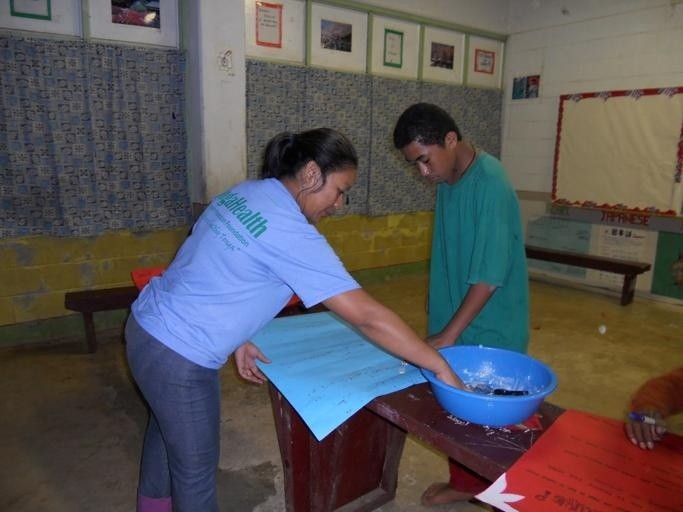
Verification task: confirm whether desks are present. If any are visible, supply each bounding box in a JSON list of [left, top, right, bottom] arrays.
[[266, 323, 566, 511]]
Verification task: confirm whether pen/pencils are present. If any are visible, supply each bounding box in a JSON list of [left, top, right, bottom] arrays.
[[628, 412, 656, 425]]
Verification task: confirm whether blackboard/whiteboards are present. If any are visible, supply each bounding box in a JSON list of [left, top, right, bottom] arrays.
[[549, 91, 683, 216]]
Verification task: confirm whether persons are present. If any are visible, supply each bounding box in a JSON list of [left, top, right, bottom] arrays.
[[671, 256, 683, 292], [393, 102, 530, 507], [125, 126, 472, 512], [625, 363, 682, 449]]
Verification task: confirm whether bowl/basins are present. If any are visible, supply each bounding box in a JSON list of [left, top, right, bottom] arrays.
[[423, 342, 558, 431]]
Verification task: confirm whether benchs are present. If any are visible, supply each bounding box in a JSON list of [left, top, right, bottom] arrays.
[[524, 243, 652, 306], [63, 286, 139, 353]]
